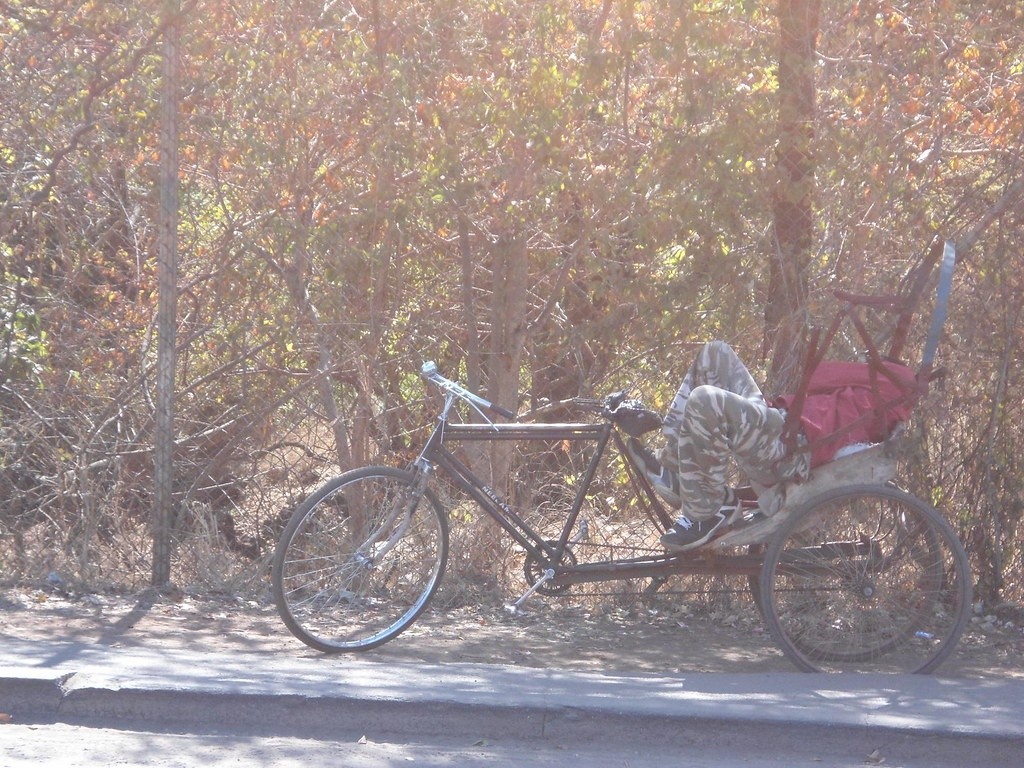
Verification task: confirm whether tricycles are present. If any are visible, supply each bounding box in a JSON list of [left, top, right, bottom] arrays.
[[269, 238, 976, 679]]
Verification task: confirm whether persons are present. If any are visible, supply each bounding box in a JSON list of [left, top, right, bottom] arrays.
[[628, 340, 917, 551]]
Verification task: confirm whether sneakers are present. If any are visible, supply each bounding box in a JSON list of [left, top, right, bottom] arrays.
[[626, 438, 681, 510], [660, 486, 740, 552]]
